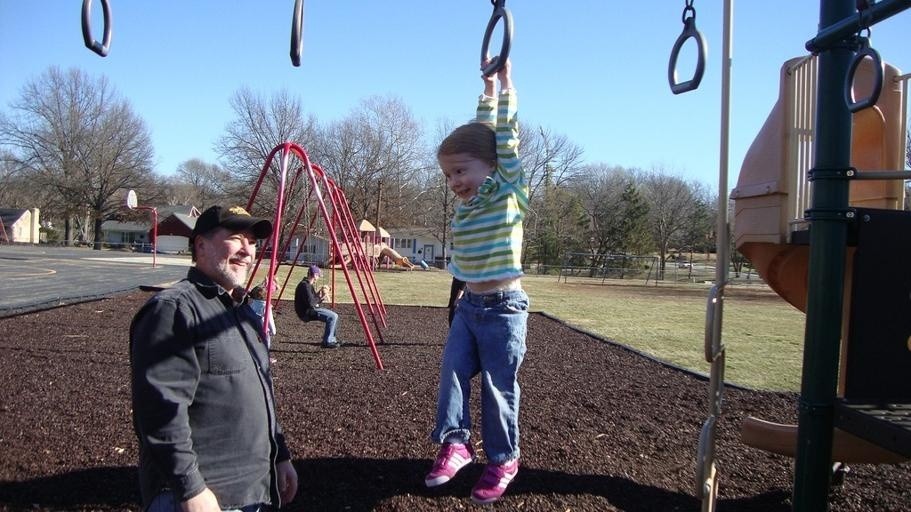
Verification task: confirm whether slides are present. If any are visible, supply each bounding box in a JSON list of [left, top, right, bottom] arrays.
[[383, 247, 415, 269], [731, 56, 911, 463]]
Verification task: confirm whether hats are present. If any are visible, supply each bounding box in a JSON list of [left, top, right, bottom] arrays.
[[188, 204, 272, 248], [308, 265, 323, 278]]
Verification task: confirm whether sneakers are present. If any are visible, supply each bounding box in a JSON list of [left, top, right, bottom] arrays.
[[470, 458, 518, 505], [424, 442, 477, 488], [320, 340, 340, 349]]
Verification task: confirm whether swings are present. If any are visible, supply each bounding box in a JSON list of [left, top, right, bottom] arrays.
[[301, 162, 333, 310]]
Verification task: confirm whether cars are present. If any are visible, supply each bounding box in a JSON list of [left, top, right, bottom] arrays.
[[678, 261, 693, 269]]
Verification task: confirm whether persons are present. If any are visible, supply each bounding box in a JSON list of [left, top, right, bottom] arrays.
[[426, 58, 529, 506], [249, 285, 278, 363], [294, 266, 340, 348], [126, 204, 297, 512]]
